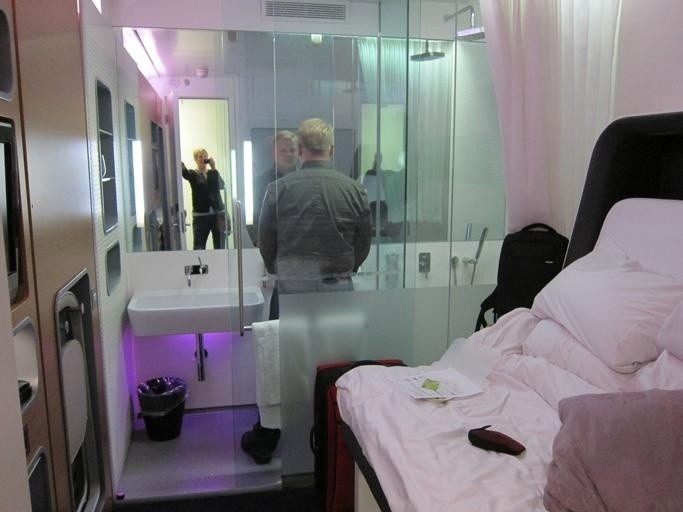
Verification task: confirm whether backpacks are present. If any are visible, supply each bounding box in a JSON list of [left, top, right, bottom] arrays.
[[474, 222, 570, 333]]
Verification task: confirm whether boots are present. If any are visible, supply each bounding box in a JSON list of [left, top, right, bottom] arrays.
[[240, 423, 281, 465]]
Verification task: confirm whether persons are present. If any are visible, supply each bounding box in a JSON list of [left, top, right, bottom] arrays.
[[181, 147, 226, 251], [241, 117, 372, 466], [244, 128, 301, 247]]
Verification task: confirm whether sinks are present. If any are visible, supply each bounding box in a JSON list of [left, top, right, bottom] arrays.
[[127, 286, 265, 337]]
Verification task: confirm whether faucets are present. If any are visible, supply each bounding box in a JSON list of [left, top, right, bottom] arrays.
[[185, 266, 193, 288]]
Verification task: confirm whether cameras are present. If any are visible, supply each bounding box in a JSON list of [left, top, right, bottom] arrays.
[[204, 159, 210, 164]]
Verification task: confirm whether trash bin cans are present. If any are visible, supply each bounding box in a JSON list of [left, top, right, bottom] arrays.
[[137, 376, 188, 441]]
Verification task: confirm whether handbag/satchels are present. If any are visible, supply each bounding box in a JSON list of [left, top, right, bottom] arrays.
[[215, 213, 231, 235]]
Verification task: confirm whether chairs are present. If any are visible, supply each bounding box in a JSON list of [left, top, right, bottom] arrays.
[[310, 104, 681, 511]]
